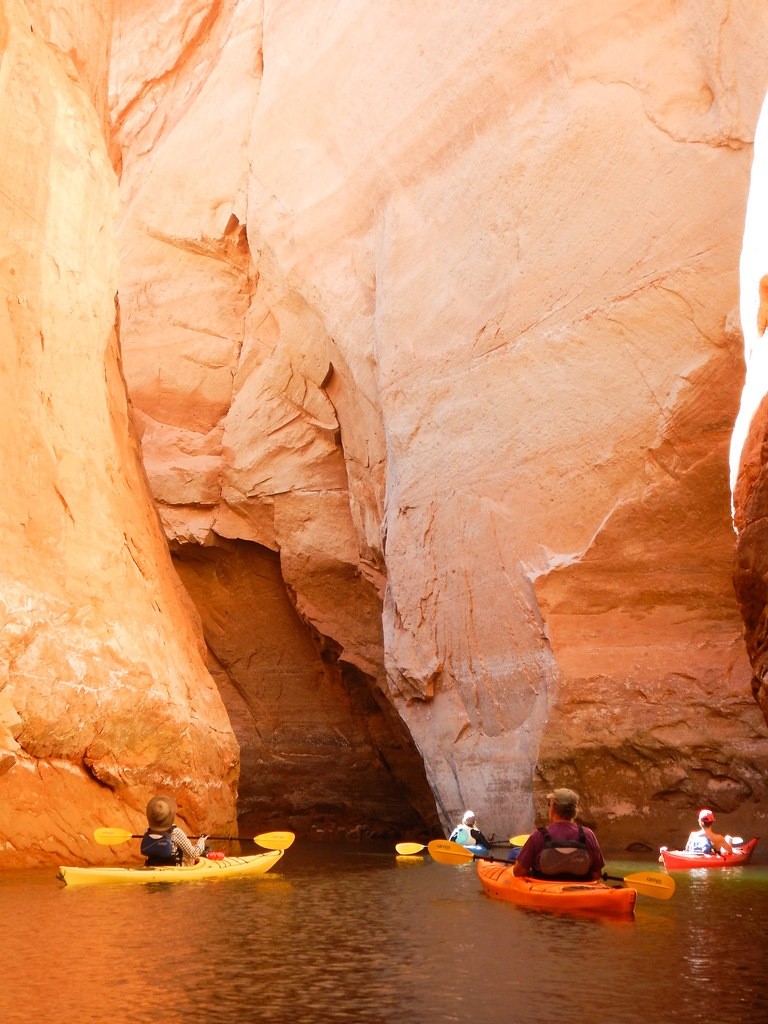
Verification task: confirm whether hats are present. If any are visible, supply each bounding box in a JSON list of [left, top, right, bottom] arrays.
[[547, 788, 578, 807], [464, 811, 475, 824], [146, 795, 177, 831], [699, 810, 715, 822]]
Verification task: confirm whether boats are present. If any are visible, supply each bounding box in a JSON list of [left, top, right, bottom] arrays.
[[660, 834, 759, 870], [475, 857, 639, 915], [462, 843, 490, 858], [56, 849, 285, 886]]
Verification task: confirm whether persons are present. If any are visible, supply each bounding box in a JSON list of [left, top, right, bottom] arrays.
[[146, 796, 209, 866], [685, 810, 732, 854], [449, 810, 491, 849], [513, 788, 606, 880]]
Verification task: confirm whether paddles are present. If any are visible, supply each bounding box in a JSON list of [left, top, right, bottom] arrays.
[[393, 832, 533, 856], [426, 838, 676, 902], [93, 827, 296, 850]]
